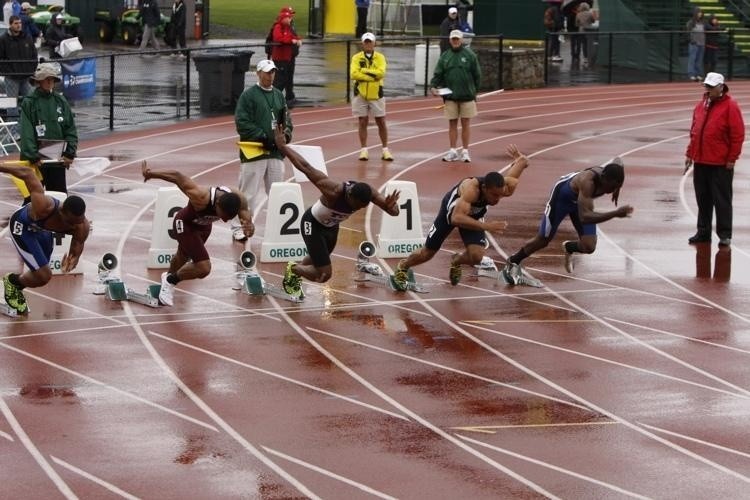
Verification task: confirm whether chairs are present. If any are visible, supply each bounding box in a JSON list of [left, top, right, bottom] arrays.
[[0, 116, 21, 156]]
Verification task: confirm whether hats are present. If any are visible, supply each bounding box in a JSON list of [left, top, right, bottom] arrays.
[[279, 7, 296, 17], [449, 30, 463, 39], [448, 7, 458, 13], [29, 63, 61, 86], [702, 72, 724, 87], [361, 33, 376, 45], [257, 59, 278, 73]]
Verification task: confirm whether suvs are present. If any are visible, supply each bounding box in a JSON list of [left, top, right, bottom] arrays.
[[28, 5, 80, 40], [96, 8, 178, 46]]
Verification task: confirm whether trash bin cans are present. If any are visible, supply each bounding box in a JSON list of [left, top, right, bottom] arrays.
[[193, 48, 256, 116]]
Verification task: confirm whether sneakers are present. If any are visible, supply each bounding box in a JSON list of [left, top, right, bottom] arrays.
[[381, 152, 394, 161], [159, 271, 176, 307], [359, 151, 369, 160], [442, 152, 459, 161], [17, 290, 28, 316], [3, 272, 20, 309], [719, 238, 730, 246], [689, 234, 711, 243], [504, 255, 520, 285], [233, 230, 248, 240], [390, 259, 410, 291], [461, 152, 471, 163], [448, 253, 462, 286], [563, 240, 575, 273], [283, 262, 306, 300]]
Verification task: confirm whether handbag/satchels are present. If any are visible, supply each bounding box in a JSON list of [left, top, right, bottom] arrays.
[[265, 28, 273, 55]]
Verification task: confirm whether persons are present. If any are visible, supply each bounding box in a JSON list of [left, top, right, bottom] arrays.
[[0, 163, 90, 315], [682, 71, 746, 247], [228, 58, 295, 243], [391, 143, 530, 292], [438, 5, 467, 58], [272, 122, 401, 301], [429, 28, 483, 164], [168, 1, 188, 57], [0, 0, 72, 144], [689, 241, 735, 350], [135, 1, 162, 58], [502, 155, 635, 288], [349, 31, 395, 162], [543, 0, 721, 84], [270, 7, 302, 106], [16, 62, 79, 276], [140, 158, 256, 307]]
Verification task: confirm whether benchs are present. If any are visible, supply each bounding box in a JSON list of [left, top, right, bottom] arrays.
[[598, 0, 750, 57]]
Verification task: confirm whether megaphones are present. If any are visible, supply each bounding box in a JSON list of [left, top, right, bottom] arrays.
[[237, 249, 257, 270], [98, 252, 118, 273], [358, 241, 377, 259]]
